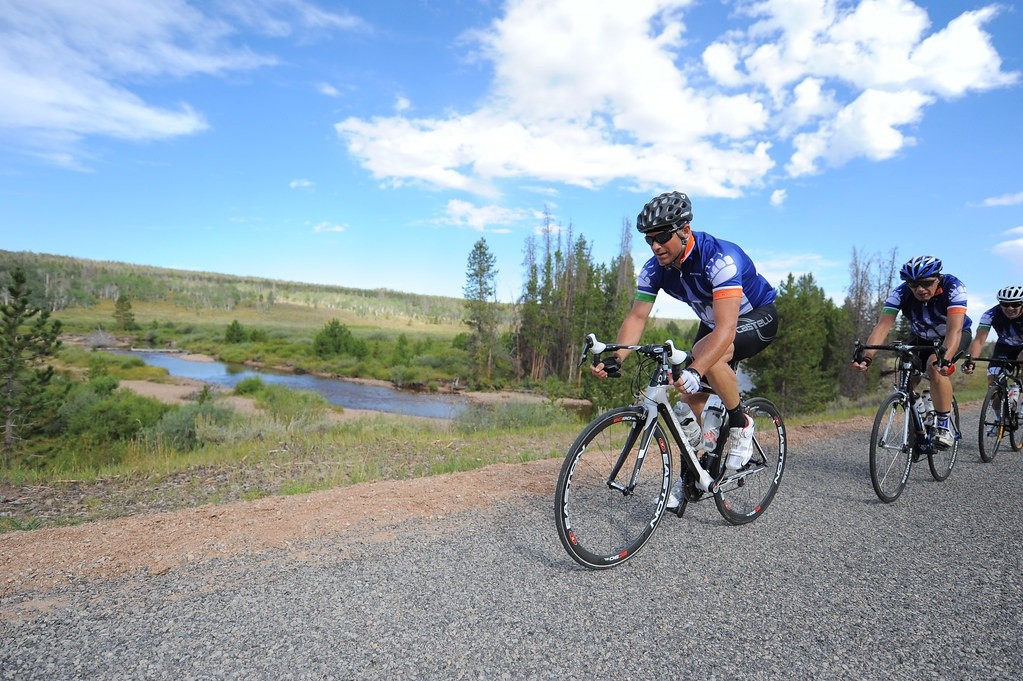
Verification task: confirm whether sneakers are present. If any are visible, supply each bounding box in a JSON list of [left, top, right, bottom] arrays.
[[653, 476, 685, 508], [725, 412, 755, 469], [986, 419, 1000, 437], [1012, 408, 1023, 426], [931, 427, 954, 447]]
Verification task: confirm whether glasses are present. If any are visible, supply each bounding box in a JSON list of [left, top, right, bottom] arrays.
[[1000, 301, 1023, 308], [645, 226, 684, 245], [907, 274, 939, 288]]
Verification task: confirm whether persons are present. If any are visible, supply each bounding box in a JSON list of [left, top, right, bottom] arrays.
[[850, 256, 972, 447], [588, 191, 779, 510], [961, 285, 1023, 436]]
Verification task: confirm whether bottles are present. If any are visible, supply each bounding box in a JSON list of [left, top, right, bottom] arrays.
[[701, 403, 724, 452], [1013, 385, 1019, 400], [913, 393, 928, 424], [922, 389, 935, 426], [672, 400, 706, 452]]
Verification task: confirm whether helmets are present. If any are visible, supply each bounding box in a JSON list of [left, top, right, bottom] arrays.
[[636, 191, 693, 233], [899, 255, 944, 280], [996, 285, 1023, 303]]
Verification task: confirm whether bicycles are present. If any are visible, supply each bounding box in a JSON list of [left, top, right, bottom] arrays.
[[963, 353, 1023, 464], [554, 332, 789, 570], [851, 337, 963, 503]]
